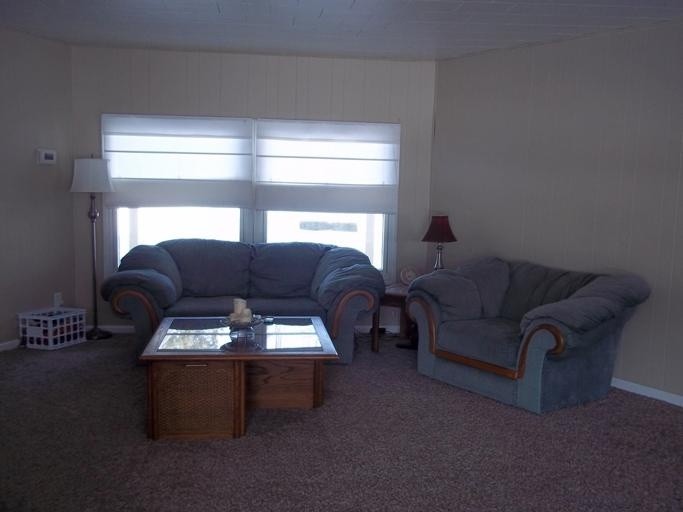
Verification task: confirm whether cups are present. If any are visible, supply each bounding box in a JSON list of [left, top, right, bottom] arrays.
[[229, 298, 253, 322]]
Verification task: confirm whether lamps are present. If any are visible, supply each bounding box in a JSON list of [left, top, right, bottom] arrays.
[[68, 154, 116, 340], [421, 216, 457, 270]]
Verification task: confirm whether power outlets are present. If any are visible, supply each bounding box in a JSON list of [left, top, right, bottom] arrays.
[[52, 291, 65, 307]]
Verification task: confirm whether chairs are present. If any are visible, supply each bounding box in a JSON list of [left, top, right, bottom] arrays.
[[405, 256, 652, 415]]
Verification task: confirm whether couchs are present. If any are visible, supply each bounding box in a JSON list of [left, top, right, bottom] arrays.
[[101, 239, 385, 365]]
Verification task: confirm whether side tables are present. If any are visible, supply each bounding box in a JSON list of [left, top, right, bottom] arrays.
[[371, 281, 410, 352]]
